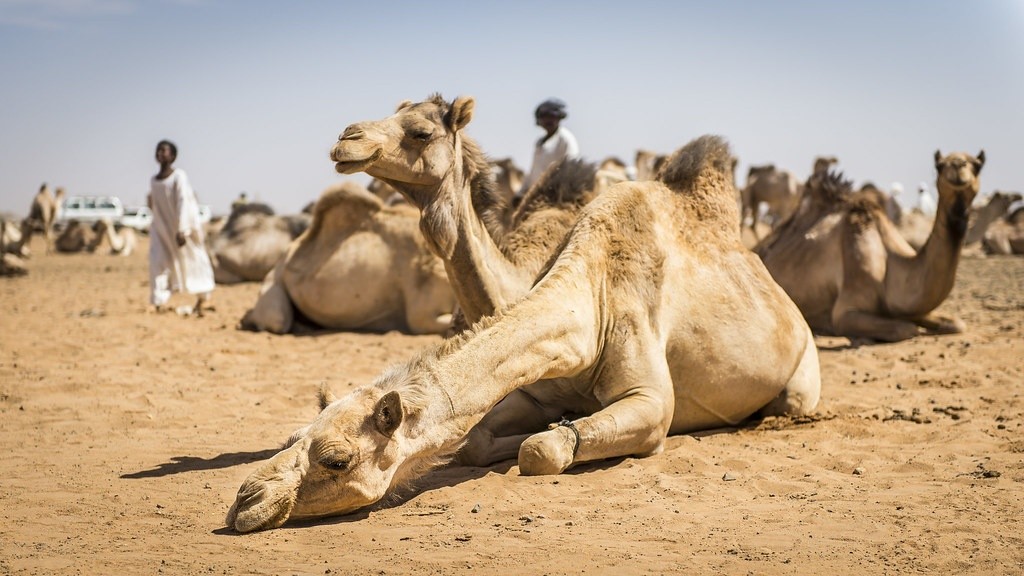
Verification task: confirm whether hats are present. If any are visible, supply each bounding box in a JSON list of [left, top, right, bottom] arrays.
[[535, 99, 567, 119]]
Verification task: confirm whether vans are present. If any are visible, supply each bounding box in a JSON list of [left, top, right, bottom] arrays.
[[57, 194, 211, 231]]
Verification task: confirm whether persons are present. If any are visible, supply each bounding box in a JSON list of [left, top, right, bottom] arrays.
[[231, 191, 248, 208], [889, 180, 939, 216], [26, 184, 65, 256], [517, 97, 578, 199], [146, 139, 216, 318]]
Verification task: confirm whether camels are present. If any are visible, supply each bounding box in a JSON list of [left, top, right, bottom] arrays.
[[205, 92, 1024, 533], [0, 183, 135, 275]]
